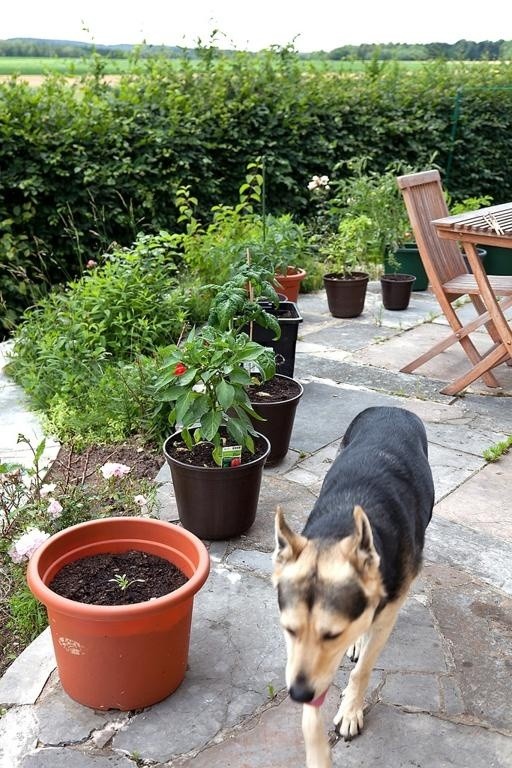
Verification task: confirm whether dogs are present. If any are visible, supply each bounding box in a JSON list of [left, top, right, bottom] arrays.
[[269, 405, 436, 768]]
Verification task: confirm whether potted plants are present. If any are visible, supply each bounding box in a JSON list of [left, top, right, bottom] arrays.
[[450, 196, 487, 273], [23, 514, 227, 717], [379, 252, 417, 311], [201, 214, 308, 469], [316, 209, 369, 318], [375, 159, 427, 291]]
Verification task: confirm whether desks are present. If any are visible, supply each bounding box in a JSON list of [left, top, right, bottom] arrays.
[[425, 192, 512, 395]]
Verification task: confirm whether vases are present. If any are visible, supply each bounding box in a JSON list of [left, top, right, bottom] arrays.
[[148, 427, 280, 548]]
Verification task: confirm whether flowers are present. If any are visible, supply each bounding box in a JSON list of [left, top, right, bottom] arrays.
[[160, 331, 273, 464]]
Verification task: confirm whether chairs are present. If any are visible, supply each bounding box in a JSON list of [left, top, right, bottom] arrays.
[[395, 170, 512, 396]]
